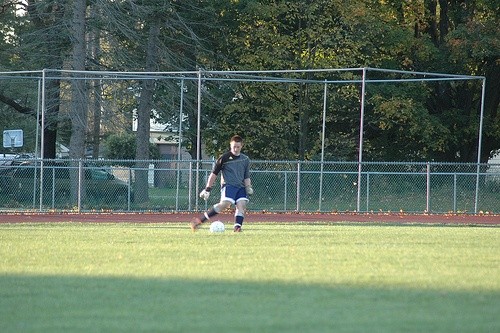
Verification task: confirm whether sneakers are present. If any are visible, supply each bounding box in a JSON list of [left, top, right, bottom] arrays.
[[234, 226, 242, 233], [191, 217, 202, 231]]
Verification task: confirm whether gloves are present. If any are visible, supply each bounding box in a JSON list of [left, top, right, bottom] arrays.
[[199, 187, 212, 200], [245, 184, 253, 195]]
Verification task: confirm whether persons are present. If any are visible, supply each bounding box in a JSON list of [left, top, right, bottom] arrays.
[[190, 136, 252, 233]]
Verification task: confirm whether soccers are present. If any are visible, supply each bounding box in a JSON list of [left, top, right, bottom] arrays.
[[210, 221, 226, 233]]
